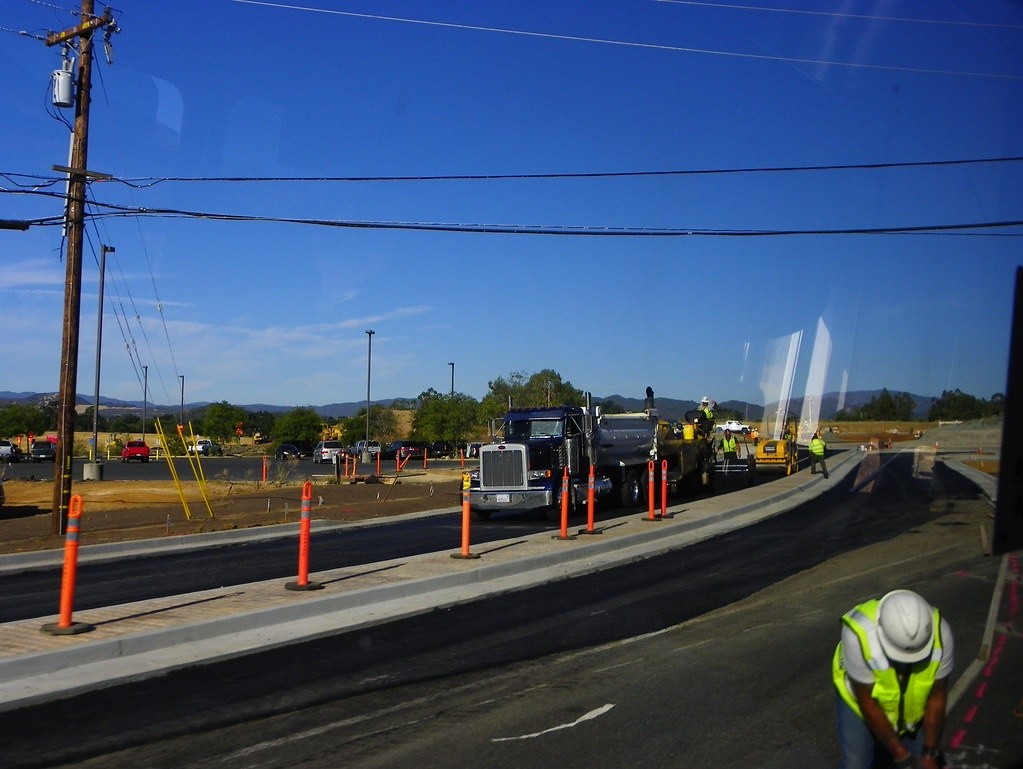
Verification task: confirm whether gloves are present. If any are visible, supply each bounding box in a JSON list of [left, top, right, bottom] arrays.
[[893, 751, 919, 769]]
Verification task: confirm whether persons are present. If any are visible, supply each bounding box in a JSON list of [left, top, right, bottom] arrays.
[[808, 433, 828, 478], [697, 396, 714, 428], [831, 589, 955, 769], [783, 428, 790, 439], [672, 423, 684, 440], [751, 428, 759, 446], [718, 429, 741, 469]]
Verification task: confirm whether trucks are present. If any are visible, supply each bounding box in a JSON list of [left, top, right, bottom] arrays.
[[188, 439, 213, 455]]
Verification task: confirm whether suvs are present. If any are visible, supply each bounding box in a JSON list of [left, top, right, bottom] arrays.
[[30, 441, 57, 464], [312, 440, 346, 464], [0, 440, 16, 461]]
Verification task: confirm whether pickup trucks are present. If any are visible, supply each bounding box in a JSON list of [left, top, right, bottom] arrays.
[[712, 420, 752, 435]]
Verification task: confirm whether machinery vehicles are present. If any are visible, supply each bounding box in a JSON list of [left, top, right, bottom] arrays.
[[746, 410, 813, 476]]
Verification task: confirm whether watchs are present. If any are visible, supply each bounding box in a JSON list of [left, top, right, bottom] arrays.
[[922, 745, 943, 757]]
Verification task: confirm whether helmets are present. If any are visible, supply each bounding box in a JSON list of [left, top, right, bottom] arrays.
[[876, 590, 934, 664], [701, 396, 709, 402], [752, 429, 754, 431], [755, 429, 757, 430]]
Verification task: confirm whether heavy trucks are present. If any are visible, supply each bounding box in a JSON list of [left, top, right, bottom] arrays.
[[458, 387, 686, 525]]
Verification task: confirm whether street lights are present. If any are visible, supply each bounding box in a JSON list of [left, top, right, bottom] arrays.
[[90, 243, 117, 463], [364, 329, 375, 452], [141, 365, 148, 442], [177, 375, 185, 426], [448, 362, 455, 398]]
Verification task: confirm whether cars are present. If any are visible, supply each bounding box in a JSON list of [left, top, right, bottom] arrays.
[[348, 438, 486, 461], [120, 440, 151, 464], [274, 445, 301, 460]]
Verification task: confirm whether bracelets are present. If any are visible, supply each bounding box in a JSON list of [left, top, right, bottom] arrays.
[[894, 752, 912, 769]]
